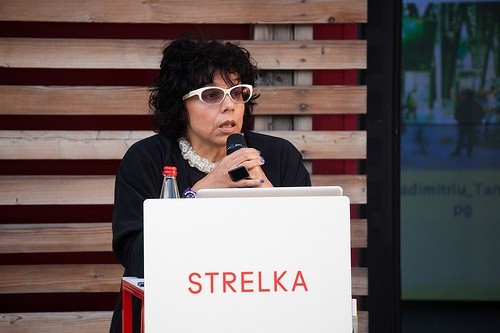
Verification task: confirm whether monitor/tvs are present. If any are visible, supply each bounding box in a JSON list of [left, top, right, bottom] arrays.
[[367, 0, 500, 333]]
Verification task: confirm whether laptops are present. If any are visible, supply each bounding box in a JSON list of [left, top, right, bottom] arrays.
[[196, 186, 343, 199]]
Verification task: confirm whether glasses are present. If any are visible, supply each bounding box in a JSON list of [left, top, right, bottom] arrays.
[[182, 84, 253, 106]]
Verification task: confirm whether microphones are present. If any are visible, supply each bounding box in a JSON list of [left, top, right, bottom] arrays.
[[226, 133, 247, 157]]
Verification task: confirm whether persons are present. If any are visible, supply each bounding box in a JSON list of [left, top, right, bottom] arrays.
[[109, 36, 311, 333]]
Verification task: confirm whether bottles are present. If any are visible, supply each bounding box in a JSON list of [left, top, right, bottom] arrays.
[[160, 167, 180, 199]]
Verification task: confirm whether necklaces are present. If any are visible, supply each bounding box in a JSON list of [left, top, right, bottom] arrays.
[[175, 138, 227, 174]]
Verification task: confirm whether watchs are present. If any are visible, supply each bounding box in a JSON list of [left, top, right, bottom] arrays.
[[181, 187, 197, 199]]
